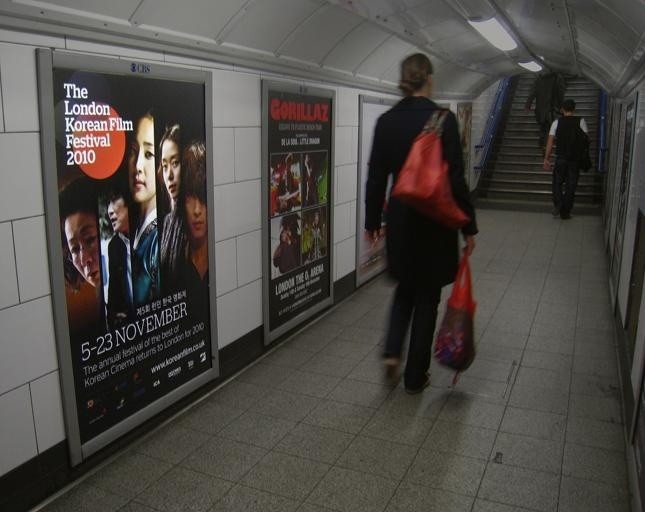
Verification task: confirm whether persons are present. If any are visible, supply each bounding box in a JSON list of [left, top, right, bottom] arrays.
[[523, 79, 565, 153], [161, 137, 209, 327], [58, 175, 103, 294], [157, 121, 199, 276], [269, 153, 327, 277], [363, 50, 481, 396], [101, 159, 128, 331], [126, 109, 160, 305], [542, 98, 592, 219]]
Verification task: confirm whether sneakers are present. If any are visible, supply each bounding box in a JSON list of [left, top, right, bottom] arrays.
[[551, 201, 572, 219], [381, 353, 398, 365], [404, 372, 431, 394]]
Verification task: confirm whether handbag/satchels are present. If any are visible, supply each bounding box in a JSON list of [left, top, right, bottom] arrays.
[[575, 126, 591, 173], [434, 249, 476, 369], [392, 130, 466, 229]]
